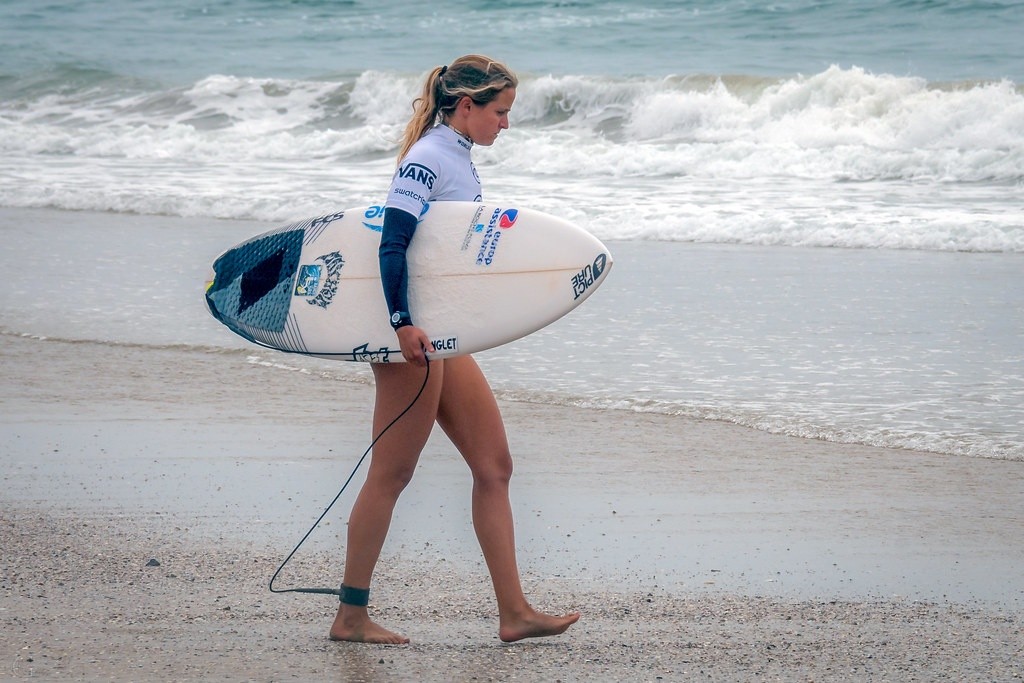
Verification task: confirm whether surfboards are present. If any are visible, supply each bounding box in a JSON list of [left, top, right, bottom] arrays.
[[199, 200, 616, 365]]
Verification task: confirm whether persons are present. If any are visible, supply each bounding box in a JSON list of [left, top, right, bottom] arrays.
[[329, 54, 581, 645]]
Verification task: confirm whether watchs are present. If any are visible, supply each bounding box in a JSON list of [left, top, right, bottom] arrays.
[[391, 311, 410, 326]]
